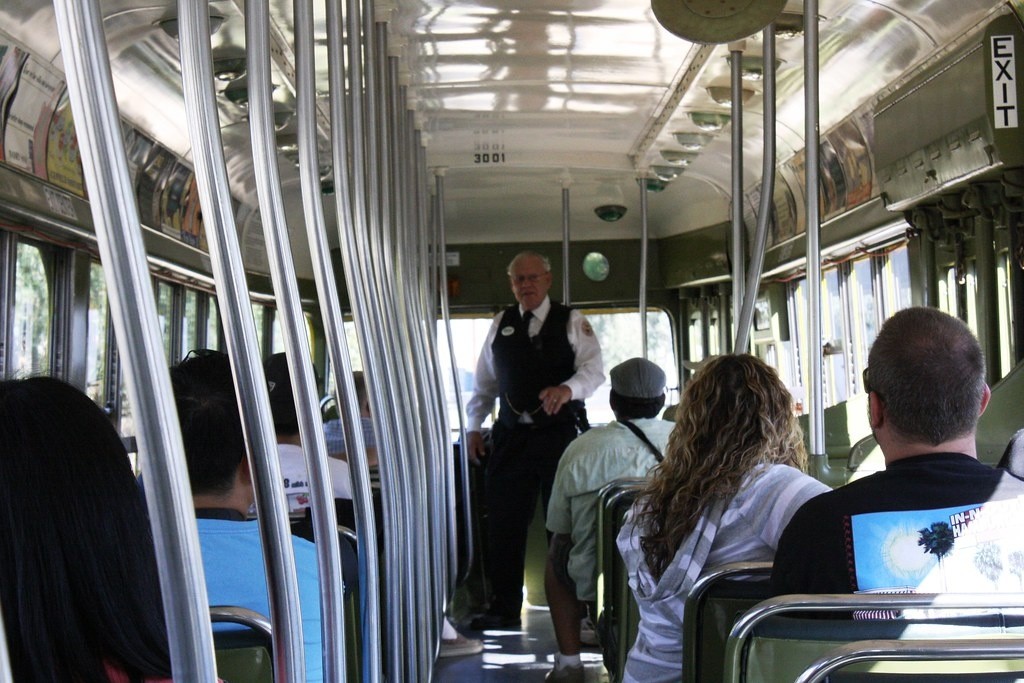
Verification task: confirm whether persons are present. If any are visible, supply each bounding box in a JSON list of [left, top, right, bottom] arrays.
[[544, 358, 676, 683], [458, 250, 606, 630], [0, 351, 485, 683], [616, 353, 835, 683], [771, 308, 1024, 621]]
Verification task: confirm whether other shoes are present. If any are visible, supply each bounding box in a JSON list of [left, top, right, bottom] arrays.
[[544, 662, 583, 683], [579, 617, 596, 642], [440, 634, 482, 657], [470, 616, 521, 630]]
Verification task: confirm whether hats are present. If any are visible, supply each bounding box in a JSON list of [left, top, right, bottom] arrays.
[[681, 355, 720, 371], [610, 357, 666, 398]]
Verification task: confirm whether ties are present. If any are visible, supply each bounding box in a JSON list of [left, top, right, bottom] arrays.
[[521, 310, 535, 337]]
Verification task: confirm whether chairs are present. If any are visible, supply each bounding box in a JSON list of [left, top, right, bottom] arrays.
[[211, 524, 356, 683], [595, 475, 1024, 683]]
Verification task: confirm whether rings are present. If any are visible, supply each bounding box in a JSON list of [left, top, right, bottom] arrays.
[[552, 400, 557, 402]]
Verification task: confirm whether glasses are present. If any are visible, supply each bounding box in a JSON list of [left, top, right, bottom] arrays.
[[510, 271, 549, 284], [178, 349, 276, 396], [863, 368, 886, 403]]
